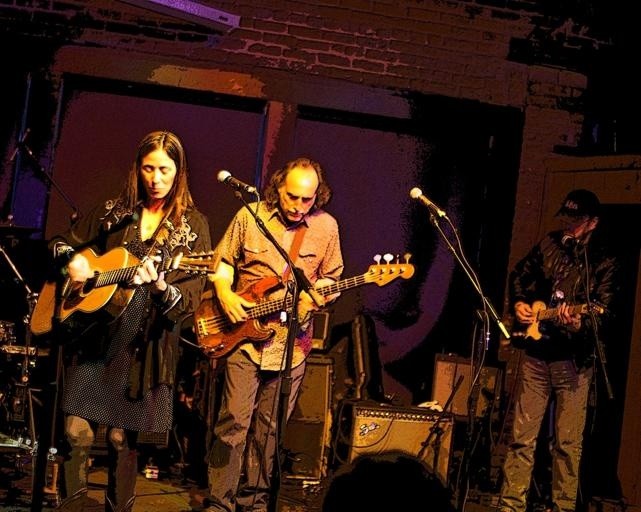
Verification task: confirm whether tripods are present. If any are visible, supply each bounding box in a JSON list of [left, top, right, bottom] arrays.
[[1, 293, 37, 446]]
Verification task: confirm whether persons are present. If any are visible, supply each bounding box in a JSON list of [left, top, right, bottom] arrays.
[[40, 128, 212, 512], [496, 187, 615, 512], [315, 448, 455, 512], [185, 157, 345, 511]]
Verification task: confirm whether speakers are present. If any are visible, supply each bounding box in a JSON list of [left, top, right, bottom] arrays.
[[348, 403, 456, 483]]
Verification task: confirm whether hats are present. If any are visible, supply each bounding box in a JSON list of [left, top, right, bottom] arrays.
[[555, 189, 599, 218]]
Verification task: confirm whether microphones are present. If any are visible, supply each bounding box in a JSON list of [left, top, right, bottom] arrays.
[[95, 213, 138, 249], [560, 233, 587, 255], [216, 169, 258, 198], [411, 187, 446, 222], [10, 125, 32, 161]]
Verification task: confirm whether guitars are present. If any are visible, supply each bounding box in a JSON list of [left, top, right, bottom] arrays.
[[501, 302, 604, 348], [31, 247, 221, 347], [195, 254, 414, 360]]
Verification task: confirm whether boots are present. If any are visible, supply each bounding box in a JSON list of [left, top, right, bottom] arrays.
[[105, 450, 138, 512], [60, 445, 89, 510]]
